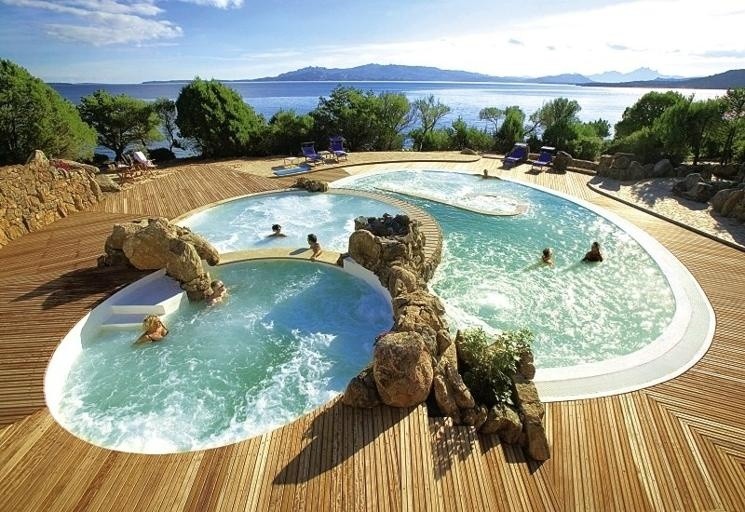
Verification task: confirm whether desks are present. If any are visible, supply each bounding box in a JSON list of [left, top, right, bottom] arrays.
[[284, 156, 300, 169], [317, 151, 331, 160]]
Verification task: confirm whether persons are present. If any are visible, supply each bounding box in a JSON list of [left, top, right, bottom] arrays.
[[582, 242, 603, 262], [269, 225, 286, 237], [307, 234, 322, 260], [542, 248, 553, 265], [294, 176, 321, 191], [135, 314, 169, 344], [206, 279, 230, 307]]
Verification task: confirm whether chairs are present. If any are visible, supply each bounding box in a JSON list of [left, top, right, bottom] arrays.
[[300, 140, 324, 167], [531, 146, 557, 172], [102, 150, 163, 188], [327, 137, 348, 161], [500, 142, 529, 170]]
